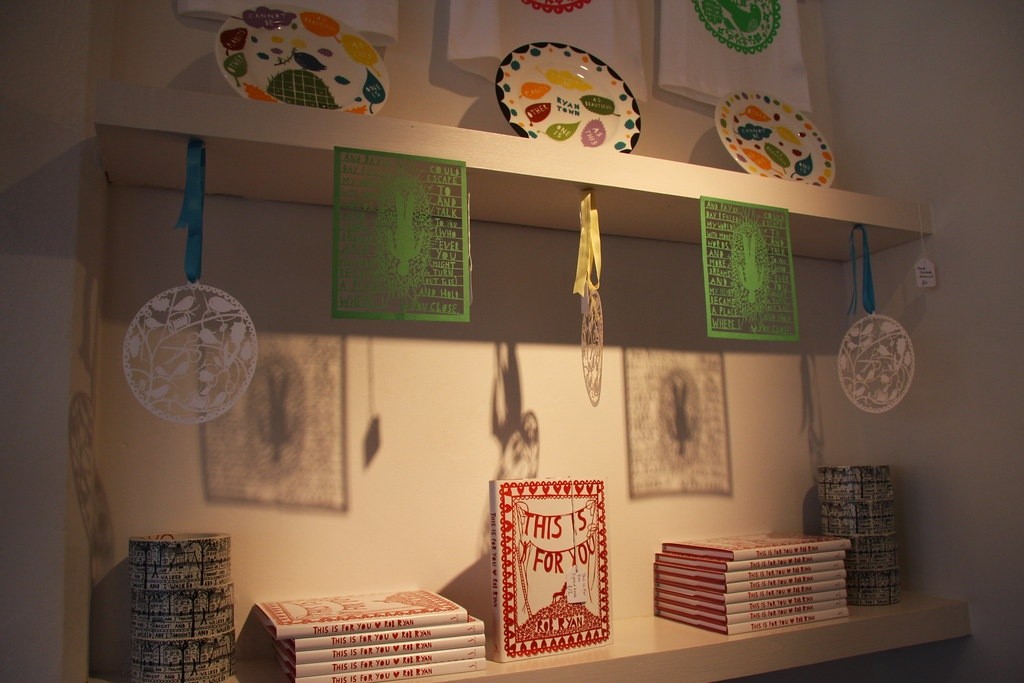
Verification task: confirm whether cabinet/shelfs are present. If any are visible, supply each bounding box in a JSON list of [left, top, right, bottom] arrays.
[[91, 89, 974, 683]]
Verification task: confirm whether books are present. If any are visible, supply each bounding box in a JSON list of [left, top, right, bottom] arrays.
[[653, 531, 851, 636], [488, 478, 616, 662], [251, 589, 487, 683]]
